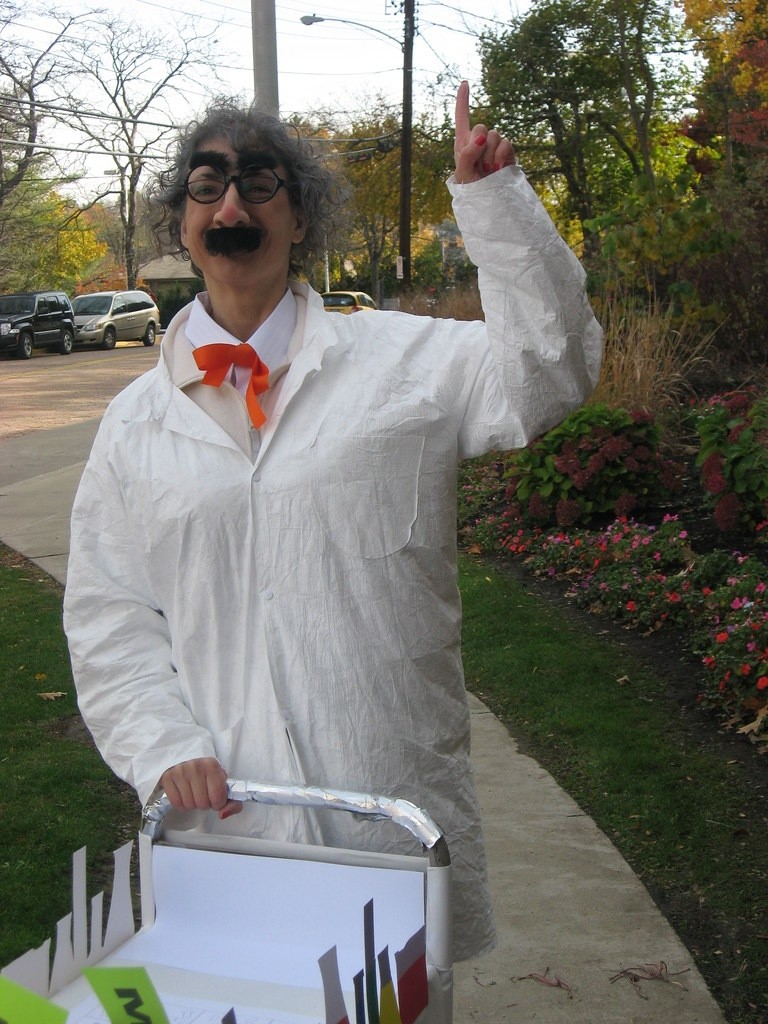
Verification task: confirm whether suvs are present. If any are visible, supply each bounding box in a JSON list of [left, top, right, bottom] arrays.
[[0, 290, 75, 359]]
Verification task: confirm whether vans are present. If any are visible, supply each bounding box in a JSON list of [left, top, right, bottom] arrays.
[[71, 289, 161, 350]]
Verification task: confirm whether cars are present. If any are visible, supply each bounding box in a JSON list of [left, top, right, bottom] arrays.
[[321, 291, 379, 317]]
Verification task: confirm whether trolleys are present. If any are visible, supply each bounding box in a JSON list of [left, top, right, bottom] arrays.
[[0, 778, 458, 1024]]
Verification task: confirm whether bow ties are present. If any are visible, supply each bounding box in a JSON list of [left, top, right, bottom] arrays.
[[191, 343, 270, 429]]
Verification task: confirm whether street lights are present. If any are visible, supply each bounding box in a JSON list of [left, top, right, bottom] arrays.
[[299, 11, 413, 295]]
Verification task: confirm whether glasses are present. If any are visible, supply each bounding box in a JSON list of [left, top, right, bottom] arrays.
[[184, 162, 291, 204]]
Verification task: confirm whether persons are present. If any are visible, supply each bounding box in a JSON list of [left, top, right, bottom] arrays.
[[60, 78, 604, 967]]
